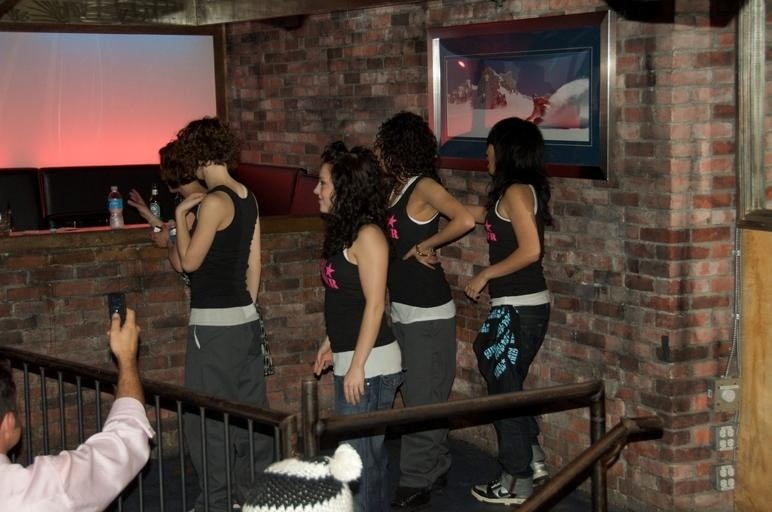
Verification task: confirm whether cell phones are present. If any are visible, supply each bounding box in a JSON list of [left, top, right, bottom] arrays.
[[108, 293, 127, 327]]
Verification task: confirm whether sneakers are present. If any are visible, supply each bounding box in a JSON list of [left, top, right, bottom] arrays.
[[469, 461, 549, 505], [392, 467, 448, 512]]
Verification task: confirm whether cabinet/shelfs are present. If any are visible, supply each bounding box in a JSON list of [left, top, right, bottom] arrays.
[[732, 208, 771, 510]]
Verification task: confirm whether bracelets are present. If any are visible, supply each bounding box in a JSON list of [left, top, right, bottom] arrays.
[[147, 213, 155, 221], [416, 244, 436, 257]]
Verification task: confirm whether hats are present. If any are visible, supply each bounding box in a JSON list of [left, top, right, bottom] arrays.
[[242, 440, 362, 512]]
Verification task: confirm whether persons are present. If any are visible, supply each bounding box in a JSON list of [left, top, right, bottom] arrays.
[[371, 109, 476, 511], [312, 140, 404, 512], [0, 308, 156, 512], [464, 116, 556, 504], [174, 115, 279, 510], [126, 139, 273, 376]]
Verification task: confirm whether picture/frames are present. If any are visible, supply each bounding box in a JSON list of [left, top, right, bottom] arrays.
[[424, 9, 620, 181]]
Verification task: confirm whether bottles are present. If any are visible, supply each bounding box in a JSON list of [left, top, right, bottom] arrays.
[[148, 183, 161, 222], [107, 186, 125, 229]]
